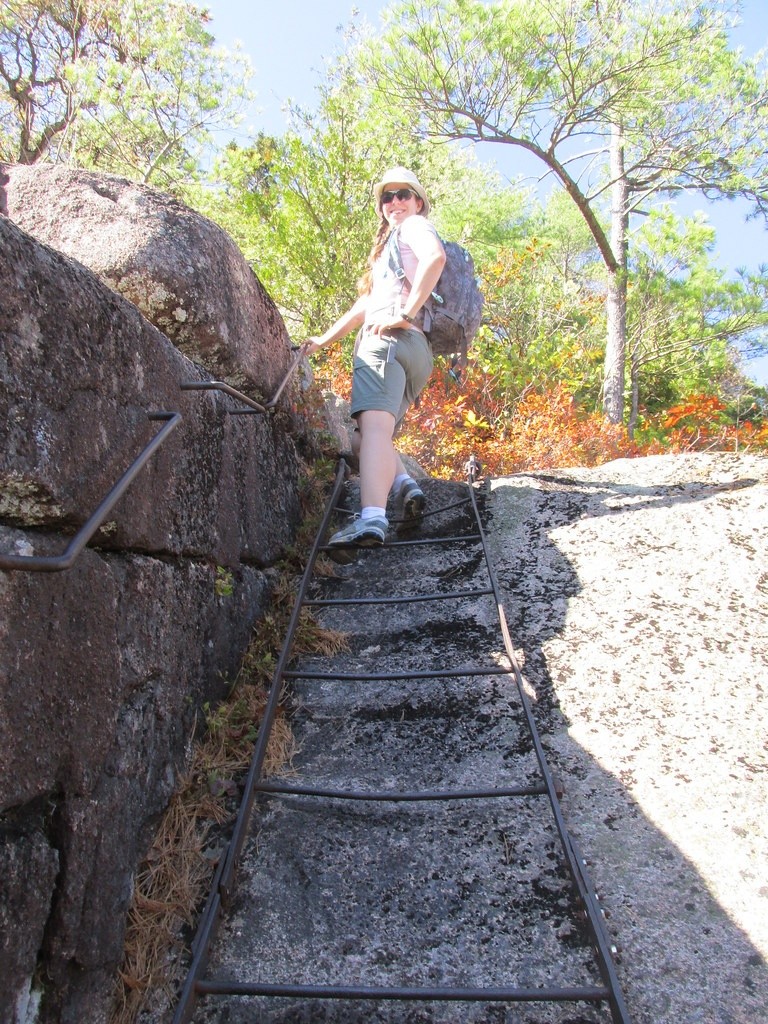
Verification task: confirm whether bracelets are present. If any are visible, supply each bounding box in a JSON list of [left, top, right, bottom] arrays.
[[401, 312, 414, 324]]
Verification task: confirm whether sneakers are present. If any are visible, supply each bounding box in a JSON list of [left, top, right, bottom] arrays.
[[388, 477, 425, 531], [325, 513, 389, 565]]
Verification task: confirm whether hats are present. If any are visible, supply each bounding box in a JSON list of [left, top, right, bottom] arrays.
[[375, 167, 430, 221]]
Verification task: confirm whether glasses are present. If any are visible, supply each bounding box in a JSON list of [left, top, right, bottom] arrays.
[[381, 188, 420, 203]]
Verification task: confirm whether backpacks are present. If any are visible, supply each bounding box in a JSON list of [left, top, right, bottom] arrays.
[[388, 225, 485, 353]]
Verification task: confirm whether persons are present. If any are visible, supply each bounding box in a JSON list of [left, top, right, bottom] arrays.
[[301, 167, 446, 544]]
